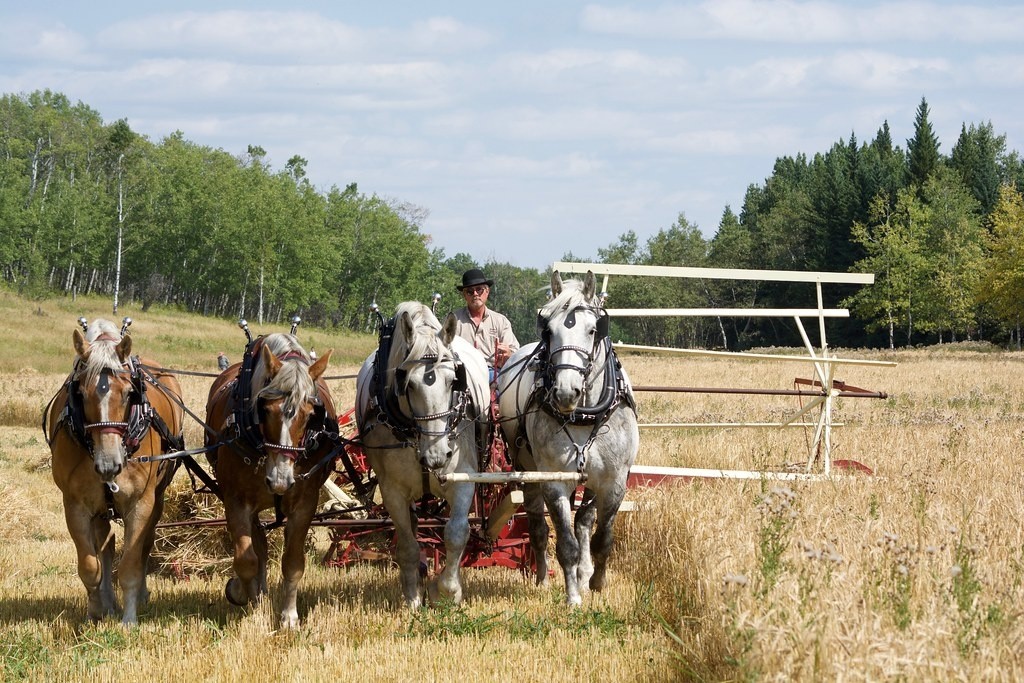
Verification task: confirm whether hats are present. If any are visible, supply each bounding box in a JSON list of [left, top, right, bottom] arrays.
[[457, 269, 494, 291]]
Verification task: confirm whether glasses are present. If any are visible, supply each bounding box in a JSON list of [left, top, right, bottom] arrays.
[[463, 287, 489, 296]]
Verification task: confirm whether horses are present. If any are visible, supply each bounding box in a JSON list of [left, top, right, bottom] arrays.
[[498, 269, 639, 615], [353, 298, 479, 615], [204, 332, 341, 633], [49, 329, 186, 631]]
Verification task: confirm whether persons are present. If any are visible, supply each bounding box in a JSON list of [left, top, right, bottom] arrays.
[[454, 269, 520, 403]]
